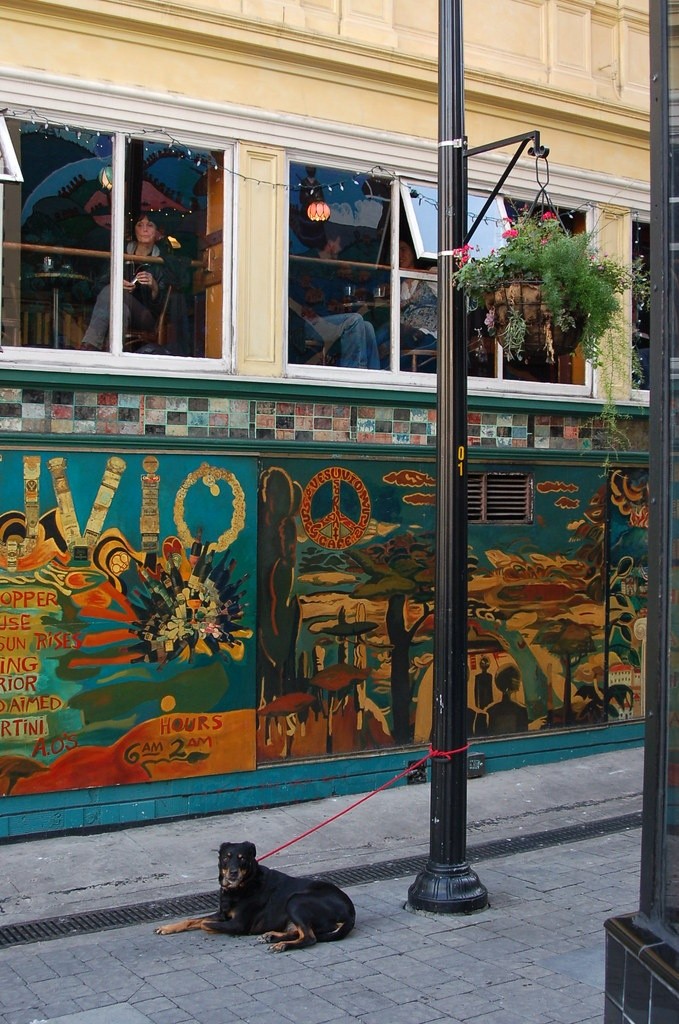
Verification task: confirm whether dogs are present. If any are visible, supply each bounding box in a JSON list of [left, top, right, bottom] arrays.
[[153, 839, 358, 954]]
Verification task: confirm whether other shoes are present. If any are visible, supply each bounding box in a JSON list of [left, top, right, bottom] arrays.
[[77, 342, 100, 351]]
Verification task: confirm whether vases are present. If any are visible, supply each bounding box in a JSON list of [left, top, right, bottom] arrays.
[[483, 282, 586, 363]]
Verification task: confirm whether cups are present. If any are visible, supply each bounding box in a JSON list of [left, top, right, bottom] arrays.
[[44, 257, 54, 271], [373, 286, 385, 297], [345, 285, 356, 296]]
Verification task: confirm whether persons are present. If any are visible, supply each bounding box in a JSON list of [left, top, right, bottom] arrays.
[[81, 211, 169, 351], [289, 221, 437, 370]]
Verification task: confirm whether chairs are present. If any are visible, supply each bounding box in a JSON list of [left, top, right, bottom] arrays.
[[1, 282, 92, 351], [105, 287, 172, 354], [288, 340, 328, 366], [400, 294, 470, 372]]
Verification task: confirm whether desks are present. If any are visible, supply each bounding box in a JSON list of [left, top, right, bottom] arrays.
[[18, 273, 94, 350], [342, 302, 389, 321]]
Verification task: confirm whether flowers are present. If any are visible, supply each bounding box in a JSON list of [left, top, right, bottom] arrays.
[[449, 183, 651, 481]]
